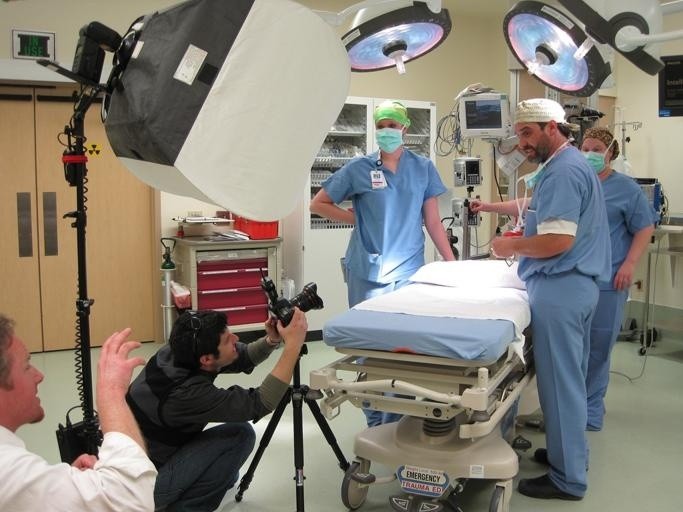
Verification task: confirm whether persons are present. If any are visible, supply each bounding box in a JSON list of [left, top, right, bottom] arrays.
[[492, 98, 609, 502], [1, 315, 157, 512], [126, 306, 308, 512], [310, 100, 455, 428], [470, 129, 660, 430]]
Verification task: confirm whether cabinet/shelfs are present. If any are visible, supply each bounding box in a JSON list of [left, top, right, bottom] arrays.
[[376, 99, 437, 263], [281, 96, 376, 341]]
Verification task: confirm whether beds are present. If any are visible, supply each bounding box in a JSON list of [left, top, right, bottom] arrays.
[[310, 260, 535, 512]]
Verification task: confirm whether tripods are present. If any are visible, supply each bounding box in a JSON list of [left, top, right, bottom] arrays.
[[234, 343, 350, 512]]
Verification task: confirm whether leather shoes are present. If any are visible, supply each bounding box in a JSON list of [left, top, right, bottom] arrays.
[[517, 473, 581, 500], [533, 448, 548, 464]]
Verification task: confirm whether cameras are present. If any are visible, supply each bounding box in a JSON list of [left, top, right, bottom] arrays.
[[251, 264, 325, 326]]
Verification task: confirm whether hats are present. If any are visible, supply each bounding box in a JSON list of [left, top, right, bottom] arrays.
[[514, 98, 565, 126], [373, 100, 411, 127], [582, 127, 619, 160]]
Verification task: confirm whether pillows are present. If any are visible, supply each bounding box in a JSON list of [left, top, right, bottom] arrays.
[[408, 260, 534, 291]]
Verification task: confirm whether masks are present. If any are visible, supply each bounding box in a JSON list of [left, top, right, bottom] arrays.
[[374, 125, 408, 154], [583, 139, 616, 173], [511, 136, 575, 233]]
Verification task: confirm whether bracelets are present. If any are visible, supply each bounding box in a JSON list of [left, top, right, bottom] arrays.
[[268, 336, 282, 343]]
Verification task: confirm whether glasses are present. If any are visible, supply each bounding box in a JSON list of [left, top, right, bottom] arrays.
[[185, 308, 202, 355]]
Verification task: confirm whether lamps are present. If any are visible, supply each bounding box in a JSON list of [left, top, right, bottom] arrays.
[[340, 0, 683, 98]]
